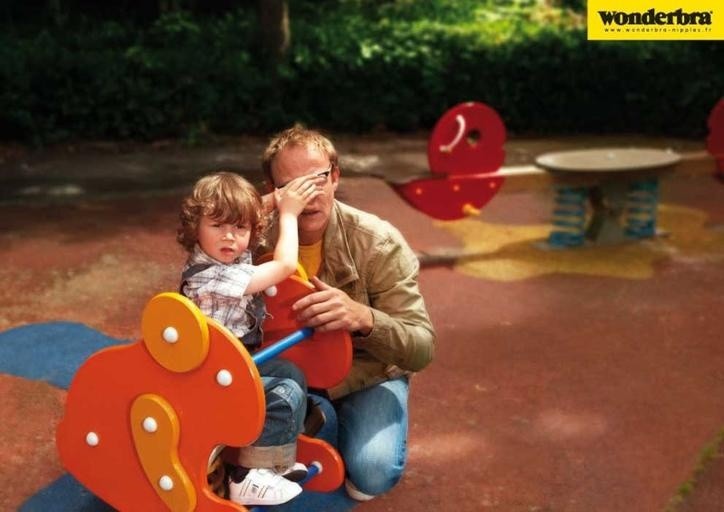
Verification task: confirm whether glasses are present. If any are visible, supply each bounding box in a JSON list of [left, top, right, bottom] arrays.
[[277, 165, 332, 194]]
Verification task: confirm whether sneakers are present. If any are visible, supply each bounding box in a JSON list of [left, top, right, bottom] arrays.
[[344, 477, 375, 502], [228, 462, 309, 507]]
